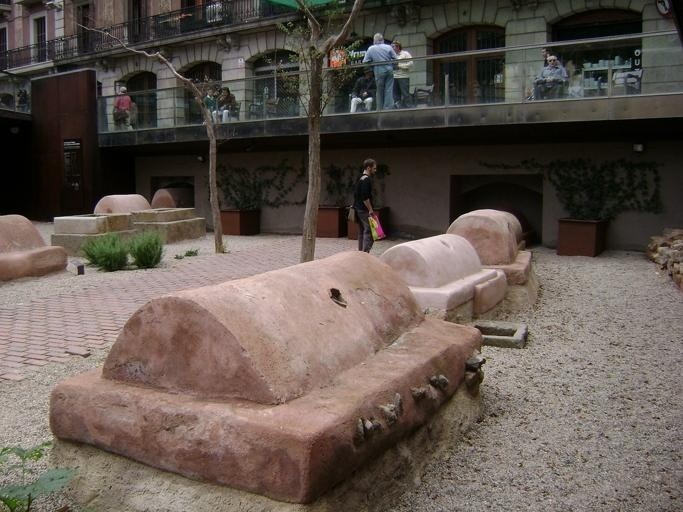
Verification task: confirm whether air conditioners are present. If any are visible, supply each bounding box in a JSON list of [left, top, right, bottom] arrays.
[[206, 2, 222, 23]]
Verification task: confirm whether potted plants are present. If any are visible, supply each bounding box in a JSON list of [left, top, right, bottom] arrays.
[[204, 160, 391, 240], [471, 152, 665, 257]]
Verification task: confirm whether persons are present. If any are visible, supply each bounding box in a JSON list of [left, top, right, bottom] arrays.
[[352, 158, 380, 253], [113, 86, 132, 132], [364, 32, 399, 111], [127, 94, 139, 130], [213, 86, 237, 125], [540, 47, 553, 67], [348, 65, 376, 113], [201, 86, 219, 125], [531, 55, 568, 101], [391, 40, 415, 109]]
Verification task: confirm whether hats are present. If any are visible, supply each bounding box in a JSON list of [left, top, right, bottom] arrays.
[[119, 87, 126, 95]]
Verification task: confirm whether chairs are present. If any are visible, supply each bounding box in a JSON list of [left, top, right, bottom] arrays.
[[348, 89, 377, 112], [228, 103, 241, 121], [248, 97, 279, 120], [532, 70, 570, 98], [402, 85, 435, 108], [598, 70, 642, 95]]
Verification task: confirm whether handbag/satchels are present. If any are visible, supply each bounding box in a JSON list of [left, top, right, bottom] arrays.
[[368, 214, 386, 240], [112, 109, 128, 122], [346, 205, 358, 223]]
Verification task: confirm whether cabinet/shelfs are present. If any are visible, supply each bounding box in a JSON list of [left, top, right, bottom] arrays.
[[581, 65, 631, 95]]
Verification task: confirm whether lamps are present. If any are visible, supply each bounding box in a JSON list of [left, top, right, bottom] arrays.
[[632, 143, 648, 153], [197, 155, 206, 163]]
